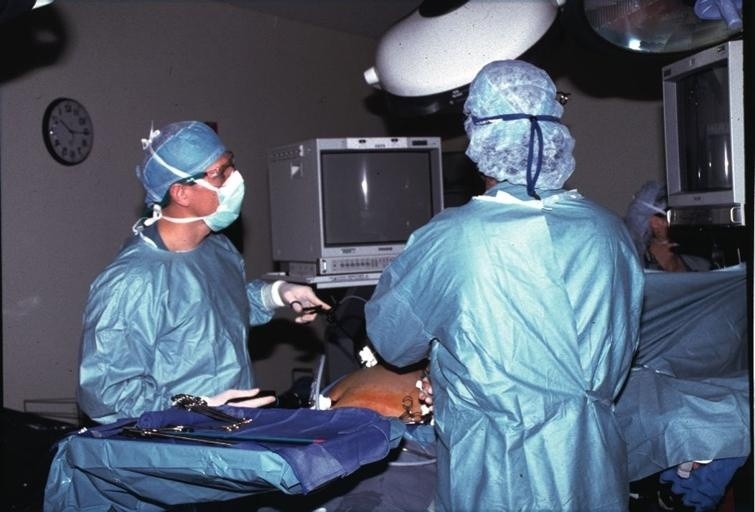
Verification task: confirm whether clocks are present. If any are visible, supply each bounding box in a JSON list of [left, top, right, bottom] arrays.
[[42, 98, 96, 166]]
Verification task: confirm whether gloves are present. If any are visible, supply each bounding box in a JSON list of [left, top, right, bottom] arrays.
[[198, 388, 276, 409], [261, 280, 330, 324]]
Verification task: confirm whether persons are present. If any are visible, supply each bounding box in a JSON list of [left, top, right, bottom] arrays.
[[628, 175, 713, 272], [75, 120, 329, 424], [364, 59, 646, 512]]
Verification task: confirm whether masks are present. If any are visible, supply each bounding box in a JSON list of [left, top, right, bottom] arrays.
[[188, 169, 246, 232]]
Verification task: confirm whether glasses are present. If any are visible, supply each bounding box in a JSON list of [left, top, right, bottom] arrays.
[[177, 156, 236, 186]]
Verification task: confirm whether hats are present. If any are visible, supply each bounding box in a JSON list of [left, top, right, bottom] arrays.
[[136, 121, 225, 206]]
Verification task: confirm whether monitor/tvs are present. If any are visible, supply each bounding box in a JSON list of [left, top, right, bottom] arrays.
[[661, 39, 744, 208], [267, 136, 445, 277]]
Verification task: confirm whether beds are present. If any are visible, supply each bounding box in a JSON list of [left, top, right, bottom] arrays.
[[42, 258, 753, 512]]
